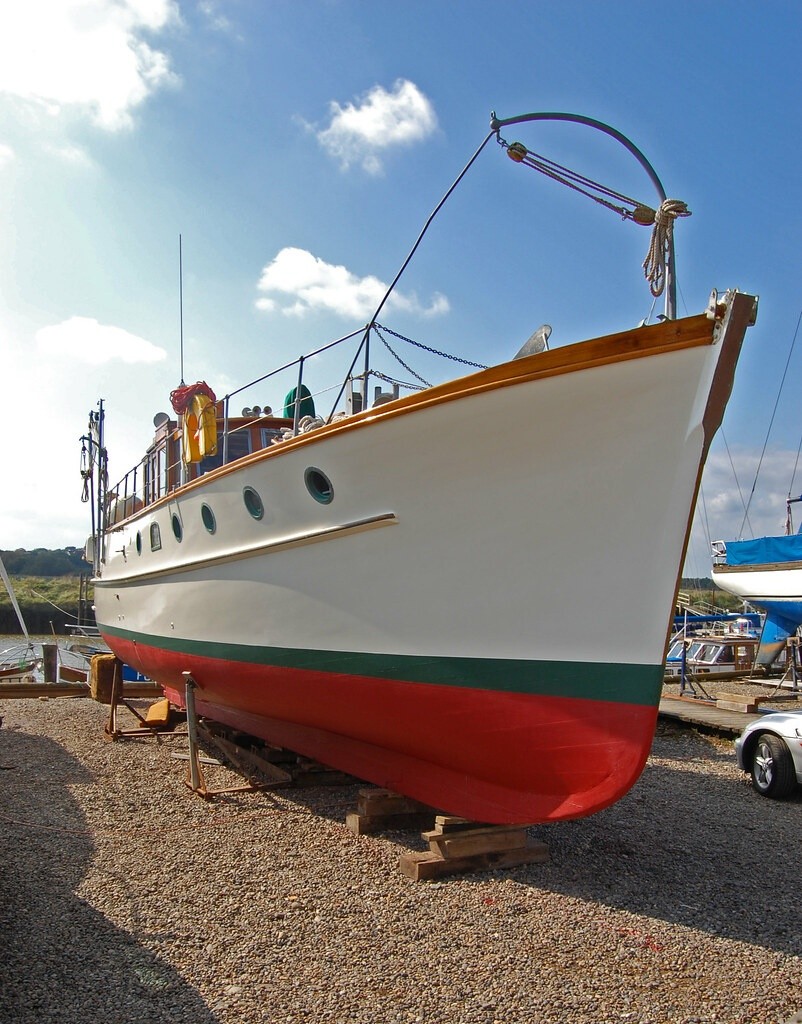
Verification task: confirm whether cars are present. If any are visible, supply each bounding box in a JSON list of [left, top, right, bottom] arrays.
[[734, 710, 802, 799]]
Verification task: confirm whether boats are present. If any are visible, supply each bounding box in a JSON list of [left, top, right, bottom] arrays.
[[0, 627, 151, 685], [661, 313, 802, 679], [85, 110, 762, 826]]
